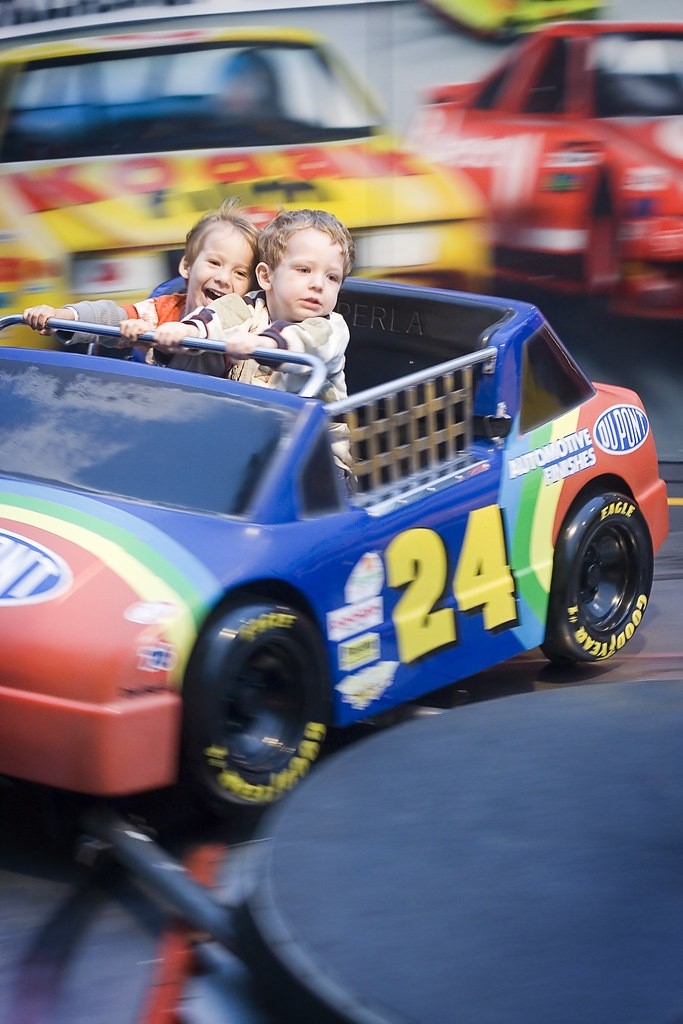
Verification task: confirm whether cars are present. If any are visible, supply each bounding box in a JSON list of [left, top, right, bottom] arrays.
[[1, 22, 502, 394], [401, 17, 683, 332], [1, 281, 671, 838]]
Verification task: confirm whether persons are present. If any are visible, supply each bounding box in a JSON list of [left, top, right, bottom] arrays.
[[218, 48, 279, 114], [22, 197, 262, 370], [153, 209, 357, 501]]
